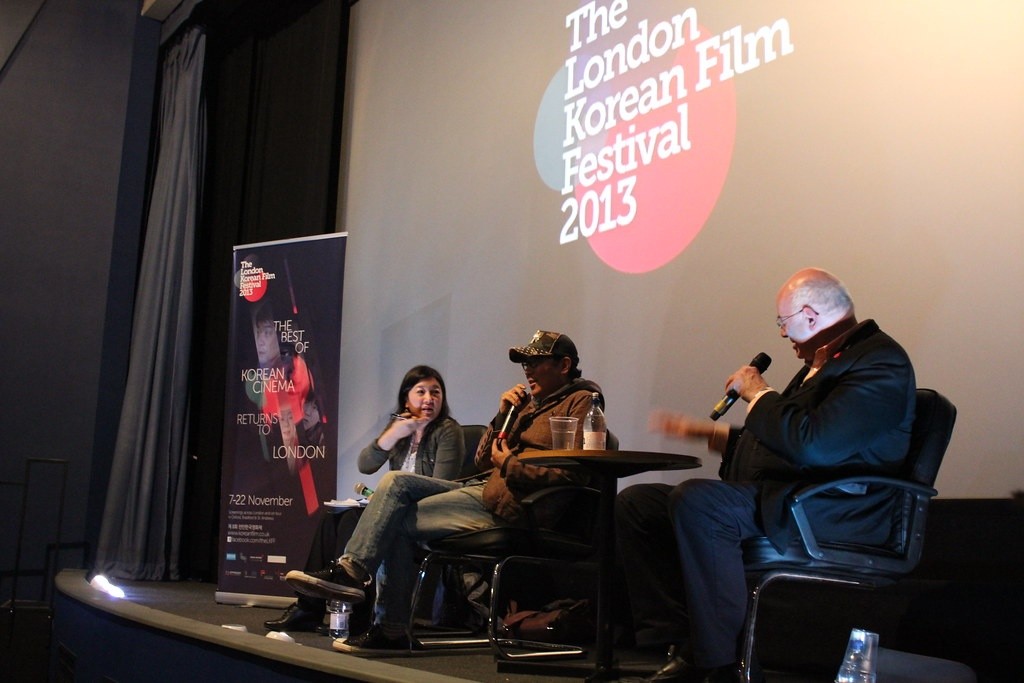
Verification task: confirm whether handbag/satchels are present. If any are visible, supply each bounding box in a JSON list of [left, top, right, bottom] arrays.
[[494, 597, 577, 644]]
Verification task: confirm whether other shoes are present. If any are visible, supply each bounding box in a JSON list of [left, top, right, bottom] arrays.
[[644, 656, 694, 683]]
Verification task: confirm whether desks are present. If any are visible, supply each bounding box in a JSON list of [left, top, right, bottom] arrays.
[[497, 449, 702, 683]]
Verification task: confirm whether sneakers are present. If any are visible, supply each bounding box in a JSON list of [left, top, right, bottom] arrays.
[[332, 622, 414, 654], [284, 558, 366, 604]]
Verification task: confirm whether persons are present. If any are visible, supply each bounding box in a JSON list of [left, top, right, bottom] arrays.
[[252, 300, 309, 426], [264, 364, 464, 633], [614, 268, 916, 683], [285, 330, 606, 655], [303, 394, 321, 433]]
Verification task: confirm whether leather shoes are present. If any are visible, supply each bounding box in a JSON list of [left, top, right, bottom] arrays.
[[263, 601, 325, 632]]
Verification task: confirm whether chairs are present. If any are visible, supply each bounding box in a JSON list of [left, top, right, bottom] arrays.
[[739, 388, 957, 683], [403, 423, 619, 655]]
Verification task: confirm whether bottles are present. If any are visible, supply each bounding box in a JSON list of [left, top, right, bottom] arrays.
[[581, 396, 606, 450], [329, 600, 351, 641]]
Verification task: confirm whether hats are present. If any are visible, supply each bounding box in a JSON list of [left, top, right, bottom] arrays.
[[508, 329, 578, 363]]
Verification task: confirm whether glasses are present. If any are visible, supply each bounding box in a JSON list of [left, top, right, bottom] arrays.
[[775, 304, 820, 328], [522, 355, 551, 374]]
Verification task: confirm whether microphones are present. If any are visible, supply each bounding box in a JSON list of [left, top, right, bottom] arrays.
[[355, 483, 375, 499], [708, 352, 772, 422], [497, 390, 528, 446]]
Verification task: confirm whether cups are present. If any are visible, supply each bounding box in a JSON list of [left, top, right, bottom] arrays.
[[549, 417, 578, 450], [837, 628, 879, 682]]
